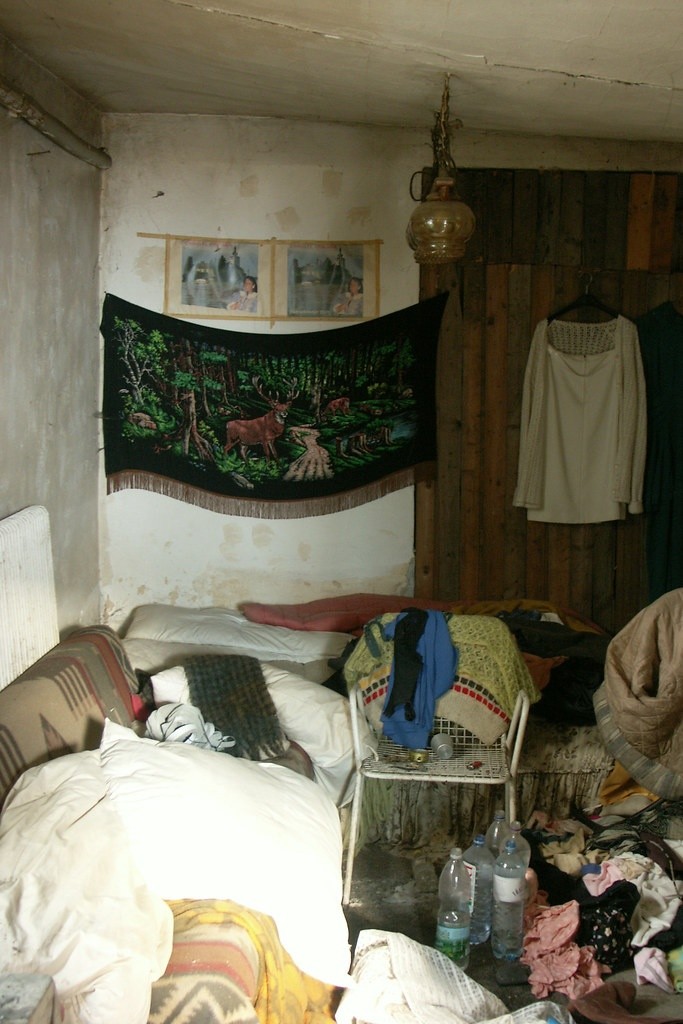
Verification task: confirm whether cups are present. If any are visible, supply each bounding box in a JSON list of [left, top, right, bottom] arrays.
[[430, 733, 454, 759]]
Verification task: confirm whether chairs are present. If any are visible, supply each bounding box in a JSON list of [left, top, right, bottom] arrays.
[[342, 613, 529, 905]]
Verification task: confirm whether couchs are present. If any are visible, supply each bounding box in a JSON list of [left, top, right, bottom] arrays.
[[0, 625, 310, 1024]]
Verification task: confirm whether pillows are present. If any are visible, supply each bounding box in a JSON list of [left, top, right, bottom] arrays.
[[97, 720, 353, 990], [149, 659, 376, 775]]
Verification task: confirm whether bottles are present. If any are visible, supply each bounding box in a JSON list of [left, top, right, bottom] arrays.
[[461, 835, 495, 945], [434, 847, 471, 971], [412, 849, 438, 892], [486, 814, 511, 859], [499, 821, 531, 873], [492, 840, 524, 960], [409, 747, 430, 763]]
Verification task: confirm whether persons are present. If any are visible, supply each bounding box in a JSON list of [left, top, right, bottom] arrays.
[[227, 276, 257, 312], [329, 278, 364, 318]]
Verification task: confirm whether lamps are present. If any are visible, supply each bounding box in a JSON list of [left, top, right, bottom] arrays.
[[404, 76, 475, 265]]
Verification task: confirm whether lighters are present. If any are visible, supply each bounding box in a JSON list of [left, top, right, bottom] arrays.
[[467, 761, 482, 770]]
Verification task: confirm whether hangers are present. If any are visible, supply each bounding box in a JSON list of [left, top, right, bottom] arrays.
[[547, 271, 617, 328]]
